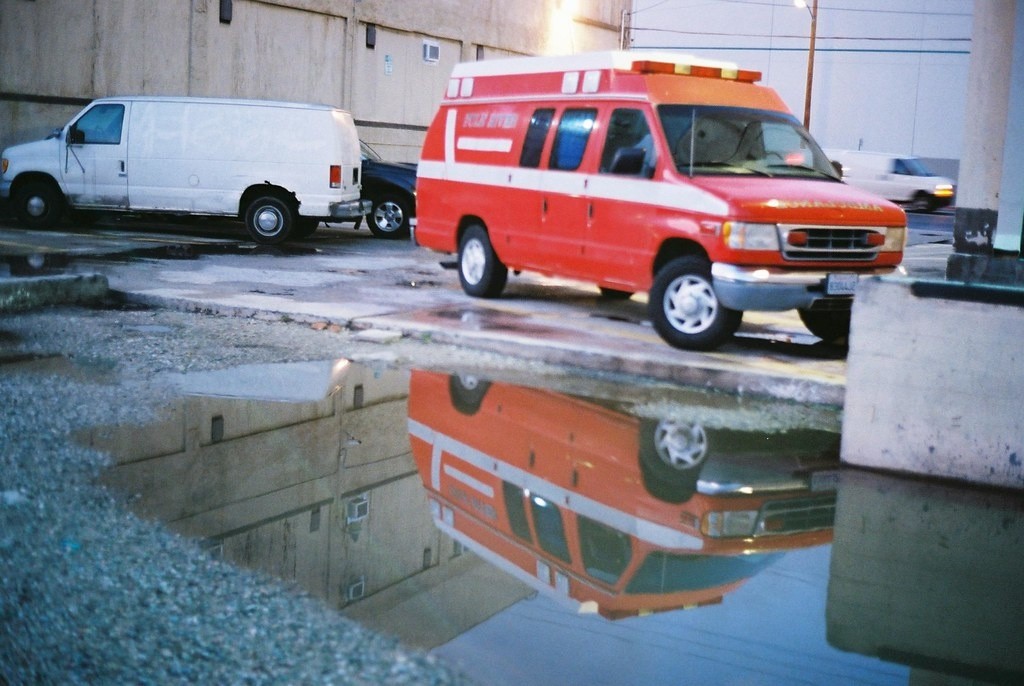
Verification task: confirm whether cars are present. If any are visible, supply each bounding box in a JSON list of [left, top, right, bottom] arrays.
[[407, 368, 838, 620], [413, 49, 908, 352]]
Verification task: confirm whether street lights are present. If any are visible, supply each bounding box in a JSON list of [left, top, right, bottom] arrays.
[[793, 0, 819, 129]]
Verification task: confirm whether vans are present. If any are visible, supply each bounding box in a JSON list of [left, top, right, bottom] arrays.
[[821, 150, 955, 212], [1, 97, 417, 244]]
